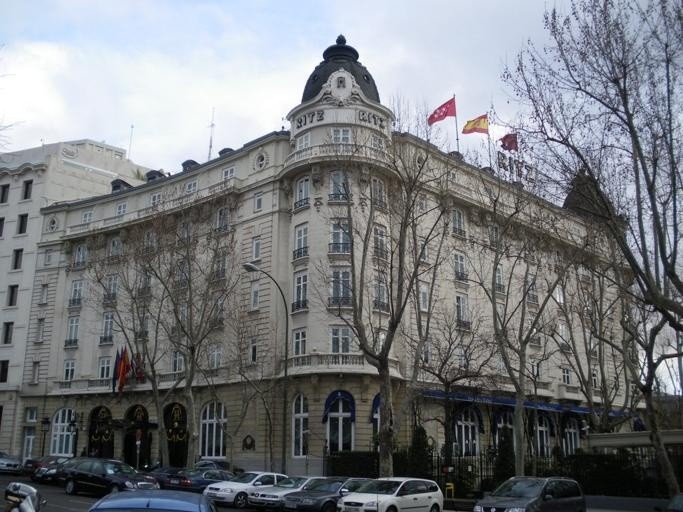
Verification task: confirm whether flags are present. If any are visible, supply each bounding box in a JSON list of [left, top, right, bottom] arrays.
[[116, 345, 129, 390], [461, 113, 488, 134], [427, 97, 456, 126], [497, 133, 518, 151], [116, 346, 122, 378], [111, 348, 119, 392]]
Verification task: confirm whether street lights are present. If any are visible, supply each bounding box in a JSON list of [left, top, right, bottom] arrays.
[[241, 262, 289, 476]]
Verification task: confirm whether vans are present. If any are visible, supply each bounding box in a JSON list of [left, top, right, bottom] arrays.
[[472, 475, 586, 511]]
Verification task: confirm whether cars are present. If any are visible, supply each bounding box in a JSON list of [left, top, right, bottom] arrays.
[[336, 476, 445, 512], [246, 474, 329, 510], [278, 475, 375, 512], [87, 488, 217, 512], [1, 452, 240, 499], [202, 470, 307, 508]]
